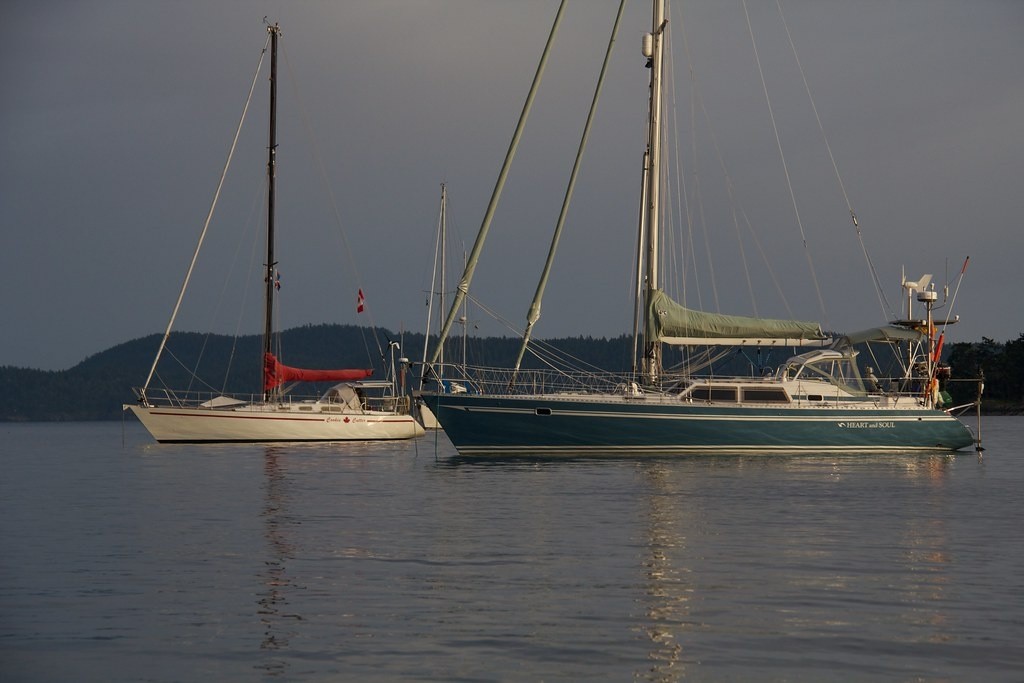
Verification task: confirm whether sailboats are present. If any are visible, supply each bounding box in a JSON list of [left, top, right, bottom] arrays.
[[123, 15, 426, 447], [410, 184, 469, 429], [398, 0, 986, 457]]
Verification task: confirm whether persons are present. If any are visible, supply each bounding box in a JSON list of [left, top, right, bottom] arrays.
[[865, 367, 878, 391], [356, 389, 367, 409]]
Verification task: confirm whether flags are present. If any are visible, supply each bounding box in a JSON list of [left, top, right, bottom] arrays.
[[357, 288, 364, 313]]
